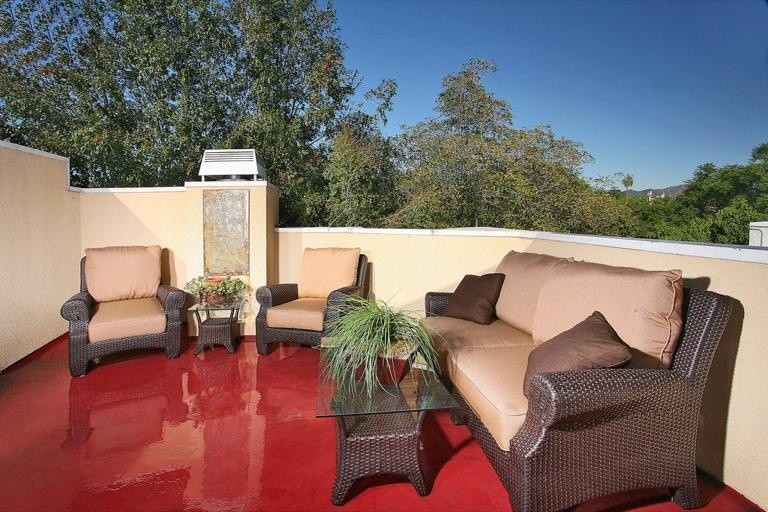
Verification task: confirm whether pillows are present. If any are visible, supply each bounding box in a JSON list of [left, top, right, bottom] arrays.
[[522, 310, 633, 400], [439, 273, 507, 325]]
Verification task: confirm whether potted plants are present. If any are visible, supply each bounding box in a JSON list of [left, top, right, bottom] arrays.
[[312, 291, 449, 386], [185, 272, 246, 305]]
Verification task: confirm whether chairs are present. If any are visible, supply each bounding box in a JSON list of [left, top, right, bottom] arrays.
[[256, 247, 368, 356], [61, 379, 190, 506], [59, 245, 187, 378]]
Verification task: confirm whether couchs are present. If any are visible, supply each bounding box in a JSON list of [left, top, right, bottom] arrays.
[[415, 248, 734, 511]]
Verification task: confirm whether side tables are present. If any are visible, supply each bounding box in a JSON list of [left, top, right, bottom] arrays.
[[187, 302, 246, 354]]
